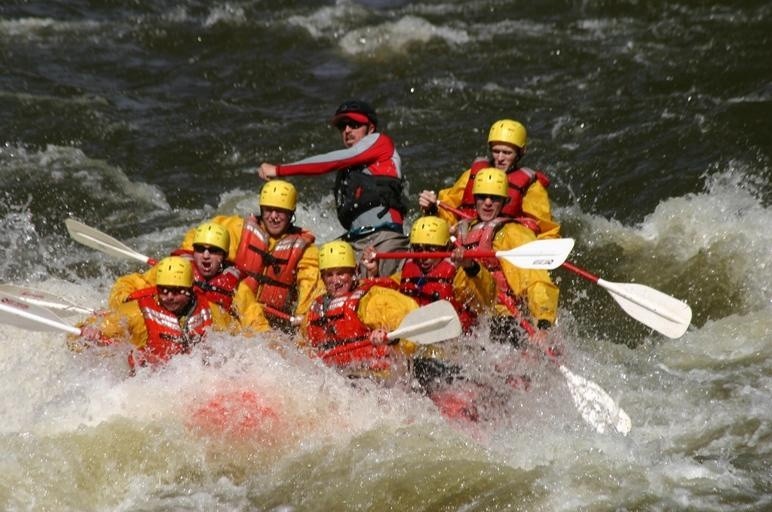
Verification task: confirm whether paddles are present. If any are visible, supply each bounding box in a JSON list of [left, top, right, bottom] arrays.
[[446, 227, 633, 439], [307, 298, 464, 361], [0, 303, 149, 363], [63, 217, 302, 329], [0, 283, 108, 319], [427, 189, 694, 340], [363, 236, 577, 272]]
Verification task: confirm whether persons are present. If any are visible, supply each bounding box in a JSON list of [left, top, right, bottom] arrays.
[[108, 223, 274, 339], [256, 99, 410, 278], [362, 214, 498, 336], [66, 254, 228, 379], [418, 166, 561, 349], [418, 117, 560, 239], [180, 178, 327, 339], [295, 240, 446, 394]]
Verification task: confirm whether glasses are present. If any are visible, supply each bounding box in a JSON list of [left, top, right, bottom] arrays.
[[337, 120, 363, 130], [192, 244, 224, 254]]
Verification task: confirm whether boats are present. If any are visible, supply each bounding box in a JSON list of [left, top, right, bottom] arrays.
[[135, 334, 559, 444]]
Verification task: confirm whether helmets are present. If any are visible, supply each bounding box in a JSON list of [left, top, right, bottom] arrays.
[[329, 100, 378, 127], [410, 119, 528, 247], [154, 180, 296, 288], [318, 240, 357, 270]]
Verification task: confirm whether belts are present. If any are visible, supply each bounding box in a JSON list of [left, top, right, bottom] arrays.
[[340, 223, 404, 241]]
